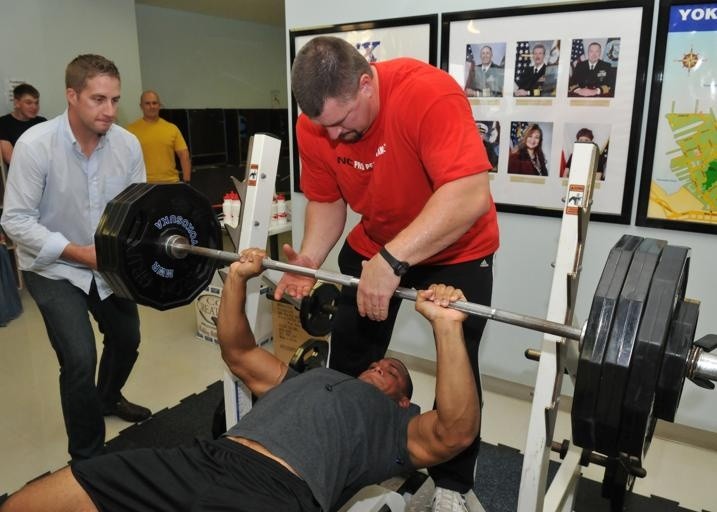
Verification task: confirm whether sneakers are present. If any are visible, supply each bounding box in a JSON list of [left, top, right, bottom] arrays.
[[430, 485, 468, 512], [103, 392, 152, 421]]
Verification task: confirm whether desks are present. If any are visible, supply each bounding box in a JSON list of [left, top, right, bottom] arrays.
[[219, 200, 291, 260]]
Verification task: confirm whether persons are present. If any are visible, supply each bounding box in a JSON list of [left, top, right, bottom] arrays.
[[570, 42, 614, 97], [125, 91, 193, 187], [271, 35, 499, 512], [465, 46, 502, 98], [0, 83, 48, 167], [361, 42, 380, 63], [0, 53, 154, 461], [563, 128, 603, 180], [476, 123, 498, 173], [0, 248, 483, 512], [513, 44, 555, 97], [508, 124, 548, 177]]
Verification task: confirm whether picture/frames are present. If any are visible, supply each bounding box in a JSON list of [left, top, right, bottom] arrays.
[[289, 14, 437, 193], [635, 0, 717, 235], [440, 1, 651, 225]]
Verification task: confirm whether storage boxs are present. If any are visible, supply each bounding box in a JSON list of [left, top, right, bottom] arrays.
[[194, 267, 273, 346], [273, 280, 342, 366]]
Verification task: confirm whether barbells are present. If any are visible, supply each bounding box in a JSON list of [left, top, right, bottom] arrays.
[[95, 182, 717, 467]]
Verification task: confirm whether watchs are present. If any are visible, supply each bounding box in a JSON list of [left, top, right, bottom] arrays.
[[378, 245, 409, 277]]
